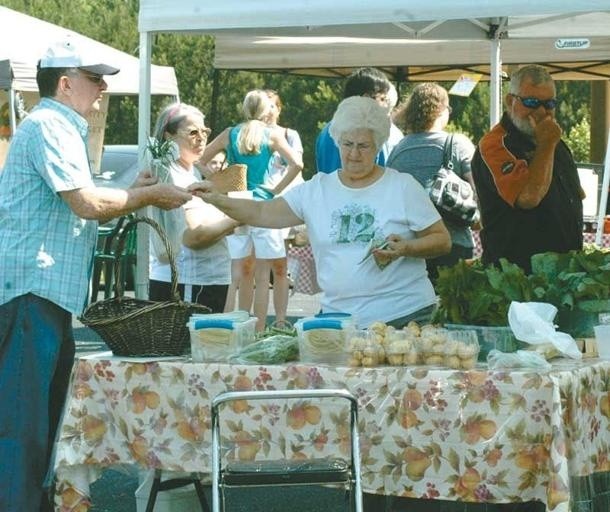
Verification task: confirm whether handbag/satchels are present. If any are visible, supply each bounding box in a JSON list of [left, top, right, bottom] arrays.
[[429, 131, 476, 223], [203, 127, 248, 193]]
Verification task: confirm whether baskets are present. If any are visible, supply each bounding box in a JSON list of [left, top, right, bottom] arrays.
[[80, 216, 212, 357]]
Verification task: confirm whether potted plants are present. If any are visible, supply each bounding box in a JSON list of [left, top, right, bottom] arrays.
[[430, 257, 527, 360], [526, 245, 609, 337]]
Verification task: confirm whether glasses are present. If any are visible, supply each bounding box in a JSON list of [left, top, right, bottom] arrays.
[[56, 70, 102, 84], [510, 94, 559, 109], [177, 127, 212, 138]]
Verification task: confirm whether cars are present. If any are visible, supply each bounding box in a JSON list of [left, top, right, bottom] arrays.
[[97, 145, 139, 292], [575, 168, 598, 214]]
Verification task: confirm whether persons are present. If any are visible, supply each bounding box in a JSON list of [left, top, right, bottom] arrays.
[[0, 48, 191, 512]]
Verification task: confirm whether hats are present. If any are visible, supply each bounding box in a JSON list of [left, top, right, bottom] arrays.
[[36, 41, 120, 76]]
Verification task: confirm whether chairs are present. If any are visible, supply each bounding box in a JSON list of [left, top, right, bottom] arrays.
[[84, 210, 139, 302], [206, 386, 366, 509]]
